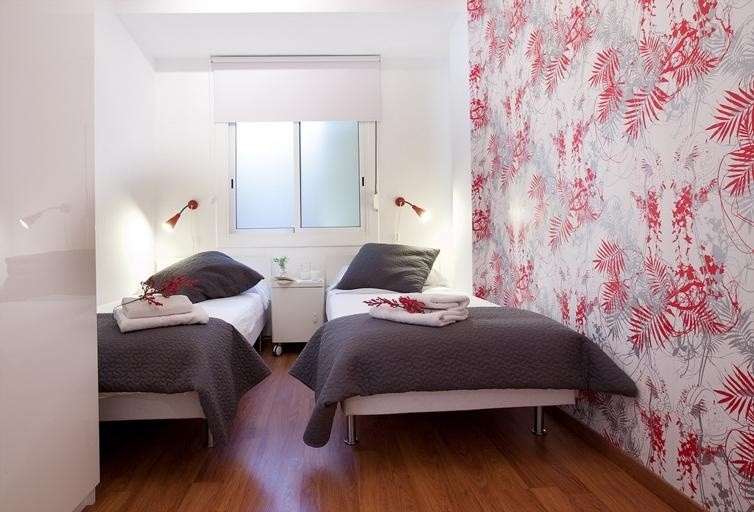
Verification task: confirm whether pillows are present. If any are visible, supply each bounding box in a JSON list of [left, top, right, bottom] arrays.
[[144, 251, 270, 310], [331, 243, 448, 293]]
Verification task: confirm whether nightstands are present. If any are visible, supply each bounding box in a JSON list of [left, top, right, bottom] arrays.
[[271, 279, 326, 356]]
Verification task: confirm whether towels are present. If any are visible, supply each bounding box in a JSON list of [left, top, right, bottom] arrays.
[[369, 304, 469, 327], [113, 305, 209, 333], [122, 295, 193, 319], [396, 293, 470, 310]]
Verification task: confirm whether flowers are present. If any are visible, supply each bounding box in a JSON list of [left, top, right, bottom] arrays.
[[363, 296, 446, 314], [114, 275, 200, 309]]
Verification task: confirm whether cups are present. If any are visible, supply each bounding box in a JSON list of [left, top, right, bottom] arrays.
[[298, 261, 322, 281]]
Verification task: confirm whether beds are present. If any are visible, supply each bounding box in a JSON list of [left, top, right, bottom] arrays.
[[97, 292, 273, 448], [326, 286, 576, 445]]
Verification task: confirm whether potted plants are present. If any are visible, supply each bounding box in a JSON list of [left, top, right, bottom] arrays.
[[273, 256, 288, 279]]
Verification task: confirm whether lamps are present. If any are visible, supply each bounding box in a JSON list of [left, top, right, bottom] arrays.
[[395, 197, 428, 220], [163, 200, 198, 232]]
